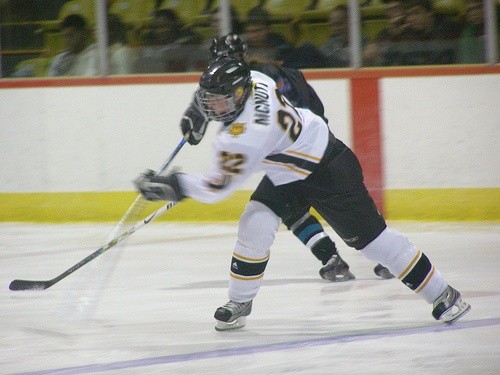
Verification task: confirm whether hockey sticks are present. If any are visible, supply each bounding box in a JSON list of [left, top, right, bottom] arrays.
[[8, 193, 186, 292], [112, 134, 191, 239]]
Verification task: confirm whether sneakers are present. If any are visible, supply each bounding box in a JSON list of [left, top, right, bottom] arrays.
[[319, 251, 355, 281], [214, 300, 252, 330], [373, 263, 395, 279], [431, 284, 471, 324]]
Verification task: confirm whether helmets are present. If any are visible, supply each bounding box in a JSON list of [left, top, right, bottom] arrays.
[[209, 33, 245, 61], [199, 55, 253, 106]]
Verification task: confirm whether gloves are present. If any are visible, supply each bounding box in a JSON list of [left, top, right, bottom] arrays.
[[181, 103, 208, 145], [132, 169, 183, 203]]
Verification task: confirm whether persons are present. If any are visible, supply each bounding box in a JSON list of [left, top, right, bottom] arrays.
[[179, 35, 396, 282], [135, 59, 470, 332], [48, 0, 500, 76]]
